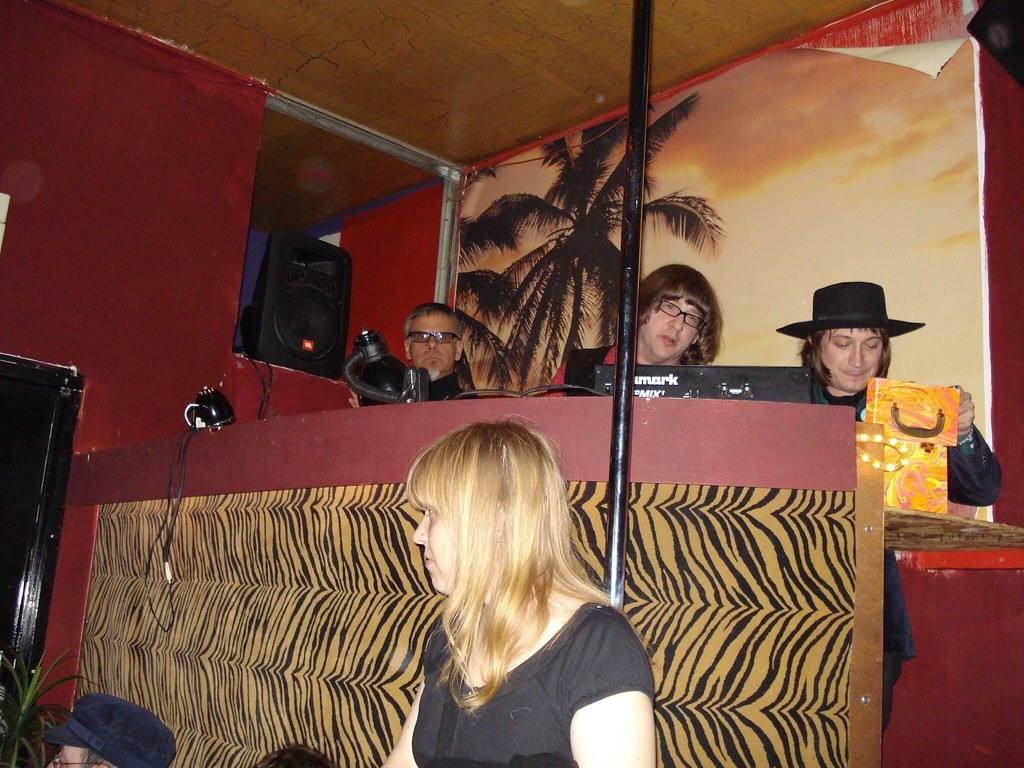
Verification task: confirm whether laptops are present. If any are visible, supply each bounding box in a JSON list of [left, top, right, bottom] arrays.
[[594, 364, 813, 404]]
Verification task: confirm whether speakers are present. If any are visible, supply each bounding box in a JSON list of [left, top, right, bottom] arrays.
[[238, 227, 352, 381]]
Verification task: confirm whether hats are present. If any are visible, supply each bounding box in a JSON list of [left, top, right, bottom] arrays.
[[44, 693, 176, 768], [776, 282, 925, 340]]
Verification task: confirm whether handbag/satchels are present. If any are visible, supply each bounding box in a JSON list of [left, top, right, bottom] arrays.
[[865, 378, 961, 513]]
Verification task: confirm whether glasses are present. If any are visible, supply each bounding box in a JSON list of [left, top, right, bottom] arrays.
[[407, 331, 459, 344], [51, 754, 110, 768], [653, 300, 707, 330]]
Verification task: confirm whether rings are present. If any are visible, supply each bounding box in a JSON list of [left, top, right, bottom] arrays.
[[953, 385, 957, 389]]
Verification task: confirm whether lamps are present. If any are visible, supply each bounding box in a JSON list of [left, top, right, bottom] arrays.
[[185, 385, 236, 431], [343, 323, 429, 407]]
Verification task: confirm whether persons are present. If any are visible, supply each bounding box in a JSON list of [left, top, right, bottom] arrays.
[[39, 692, 338, 768], [348, 302, 473, 408], [776, 281, 1002, 731], [545, 263, 722, 398], [380, 417, 655, 768]]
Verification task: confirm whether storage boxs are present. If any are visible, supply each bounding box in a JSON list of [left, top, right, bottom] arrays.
[[864, 377, 961, 514]]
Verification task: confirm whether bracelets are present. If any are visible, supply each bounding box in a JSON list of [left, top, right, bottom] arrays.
[[957, 425, 974, 444]]
